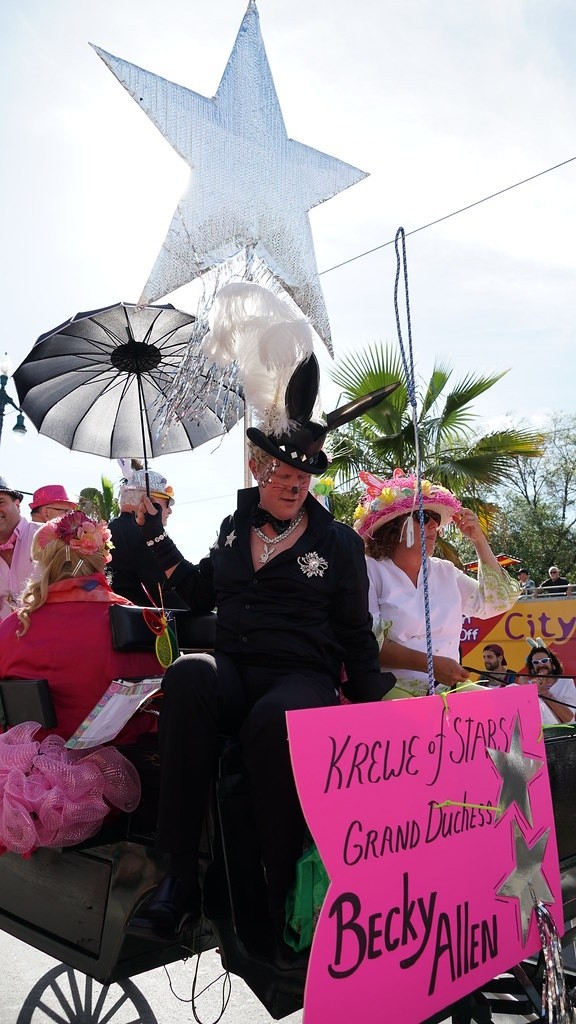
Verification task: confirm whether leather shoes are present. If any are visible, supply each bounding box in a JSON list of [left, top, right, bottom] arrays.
[[123, 872, 204, 944], [204, 850, 298, 971]]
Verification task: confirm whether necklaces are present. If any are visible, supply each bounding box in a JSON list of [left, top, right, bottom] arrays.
[[253, 501, 306, 563]]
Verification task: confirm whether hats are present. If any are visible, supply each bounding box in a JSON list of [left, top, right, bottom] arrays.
[[483, 644, 506, 667], [29, 485, 78, 510], [0, 477, 23, 502], [352, 468, 462, 543], [516, 570, 528, 575], [202, 281, 401, 476], [117, 458, 175, 506]]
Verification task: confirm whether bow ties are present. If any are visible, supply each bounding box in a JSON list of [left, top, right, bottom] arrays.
[[0, 532, 18, 551], [250, 506, 292, 536]]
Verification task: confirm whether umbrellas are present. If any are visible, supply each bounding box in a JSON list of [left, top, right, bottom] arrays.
[[11, 298, 245, 497]]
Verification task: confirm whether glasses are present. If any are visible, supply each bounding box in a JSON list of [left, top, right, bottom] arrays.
[[41, 506, 71, 514], [397, 508, 442, 526], [549, 571, 558, 574], [531, 657, 551, 665], [149, 492, 171, 508], [517, 573, 523, 576]]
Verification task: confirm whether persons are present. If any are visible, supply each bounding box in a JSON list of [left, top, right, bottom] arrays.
[[475, 644, 520, 688], [105, 458, 200, 605], [27, 485, 79, 523], [516, 569, 535, 595], [0, 510, 185, 747], [352, 468, 572, 730], [533, 566, 573, 598], [0, 472, 45, 623], [518, 637, 576, 725], [137, 420, 397, 962]]
